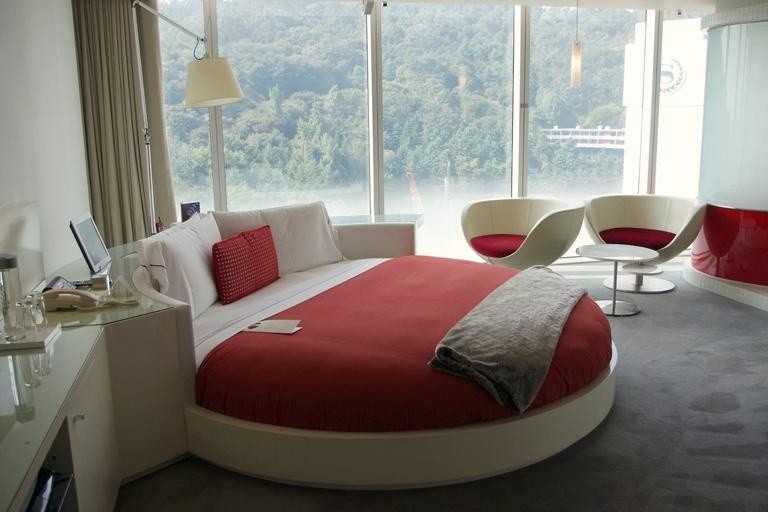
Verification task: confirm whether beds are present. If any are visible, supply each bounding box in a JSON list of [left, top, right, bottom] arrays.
[[24, 213, 619, 493]]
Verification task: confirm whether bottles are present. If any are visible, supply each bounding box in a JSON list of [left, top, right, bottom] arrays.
[[1, 254, 26, 342], [7, 355, 36, 422]]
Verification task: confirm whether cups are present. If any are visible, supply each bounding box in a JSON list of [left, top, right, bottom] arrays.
[[30, 347, 57, 387], [14, 294, 47, 343]]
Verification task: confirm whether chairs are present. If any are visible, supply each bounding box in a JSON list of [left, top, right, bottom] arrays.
[[458, 194, 706, 295]]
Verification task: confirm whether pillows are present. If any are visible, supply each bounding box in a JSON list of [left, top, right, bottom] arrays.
[[153, 200, 347, 321]]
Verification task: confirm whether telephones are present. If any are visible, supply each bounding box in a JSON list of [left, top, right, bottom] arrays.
[[42, 277, 104, 310]]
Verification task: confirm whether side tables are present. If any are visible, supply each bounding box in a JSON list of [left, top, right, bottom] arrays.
[[575, 244, 660, 318]]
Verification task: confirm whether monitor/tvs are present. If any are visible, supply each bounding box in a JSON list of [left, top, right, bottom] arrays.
[[69, 214, 112, 273]]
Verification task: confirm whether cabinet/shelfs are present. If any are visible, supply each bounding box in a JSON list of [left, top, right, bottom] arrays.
[[0, 327, 125, 511]]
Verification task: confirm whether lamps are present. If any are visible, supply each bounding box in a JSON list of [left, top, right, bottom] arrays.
[[131, 1, 246, 234], [570, 0, 583, 90]]
